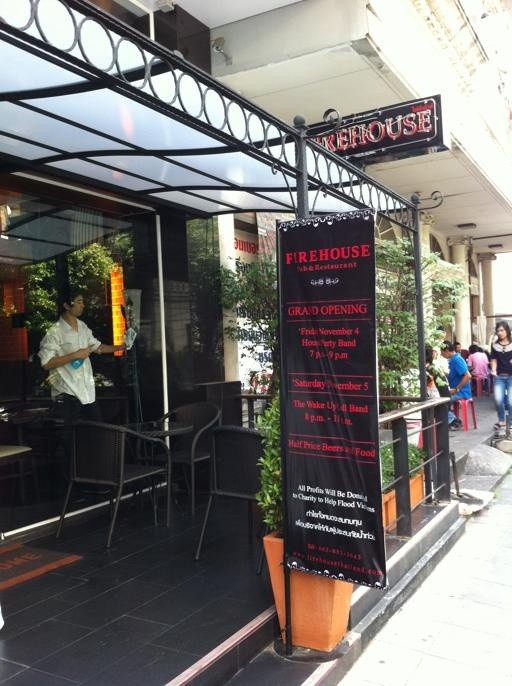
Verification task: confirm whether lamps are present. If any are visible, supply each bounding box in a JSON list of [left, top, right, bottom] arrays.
[[457, 223, 476, 230], [488, 244, 503, 249]]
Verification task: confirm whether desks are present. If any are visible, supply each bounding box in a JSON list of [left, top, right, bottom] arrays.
[[8, 412, 40, 499], [122, 422, 193, 527], [232, 394, 273, 428]]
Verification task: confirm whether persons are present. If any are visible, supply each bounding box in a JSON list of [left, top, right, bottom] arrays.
[[490, 321, 511, 434], [39, 286, 129, 505], [391, 341, 489, 430]]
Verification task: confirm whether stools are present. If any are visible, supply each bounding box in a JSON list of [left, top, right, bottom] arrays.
[[453, 398, 476, 431], [471, 375, 491, 397]]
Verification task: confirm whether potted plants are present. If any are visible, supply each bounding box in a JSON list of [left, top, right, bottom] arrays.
[[380, 444, 427, 532], [254, 391, 353, 653]]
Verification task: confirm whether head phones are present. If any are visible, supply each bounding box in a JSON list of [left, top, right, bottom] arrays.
[[67, 292, 75, 306]]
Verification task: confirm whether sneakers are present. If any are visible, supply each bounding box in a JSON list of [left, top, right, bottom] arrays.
[[448, 419, 462, 430]]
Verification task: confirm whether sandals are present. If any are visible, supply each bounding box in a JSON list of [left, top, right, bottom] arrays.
[[494, 421, 504, 430]]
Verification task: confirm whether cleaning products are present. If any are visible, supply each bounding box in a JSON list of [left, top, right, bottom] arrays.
[[70, 342, 99, 370]]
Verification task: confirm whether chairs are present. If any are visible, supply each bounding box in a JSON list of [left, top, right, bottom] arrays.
[[0, 445, 39, 529], [45, 419, 175, 548], [195, 426, 271, 575], [137, 402, 223, 515]]
